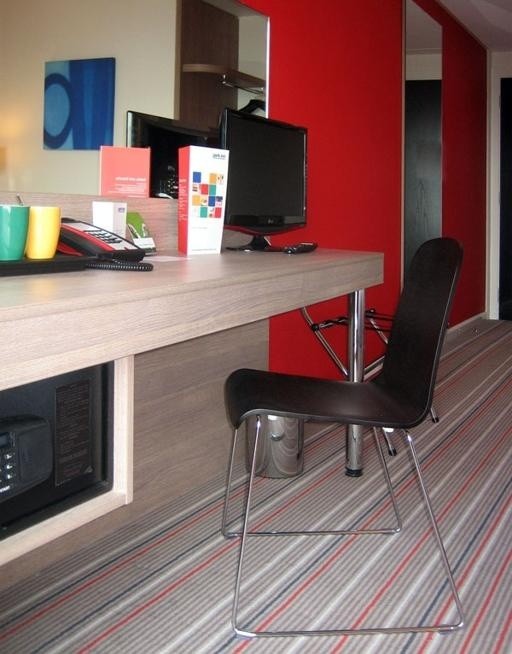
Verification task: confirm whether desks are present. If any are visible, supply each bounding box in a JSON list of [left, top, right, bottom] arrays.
[[0, 243, 387, 481]]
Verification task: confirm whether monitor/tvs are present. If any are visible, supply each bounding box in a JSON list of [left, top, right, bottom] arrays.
[[217, 105, 308, 252], [125, 110, 215, 198]]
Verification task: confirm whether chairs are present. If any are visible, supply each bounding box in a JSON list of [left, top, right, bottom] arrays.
[[219, 229, 469, 641]]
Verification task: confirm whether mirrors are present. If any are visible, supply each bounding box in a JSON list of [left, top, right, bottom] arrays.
[[1, 0, 269, 197], [400, 0, 443, 301]]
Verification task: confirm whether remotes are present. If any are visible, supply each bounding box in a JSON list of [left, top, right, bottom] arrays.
[[285, 240, 317, 253]]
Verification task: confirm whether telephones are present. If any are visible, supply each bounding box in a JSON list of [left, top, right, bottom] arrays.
[[57, 218, 145, 265]]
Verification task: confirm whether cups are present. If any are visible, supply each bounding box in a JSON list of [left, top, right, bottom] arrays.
[[0, 204, 62, 260]]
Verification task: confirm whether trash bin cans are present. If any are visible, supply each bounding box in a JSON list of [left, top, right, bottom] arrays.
[[246, 414, 305, 478]]
